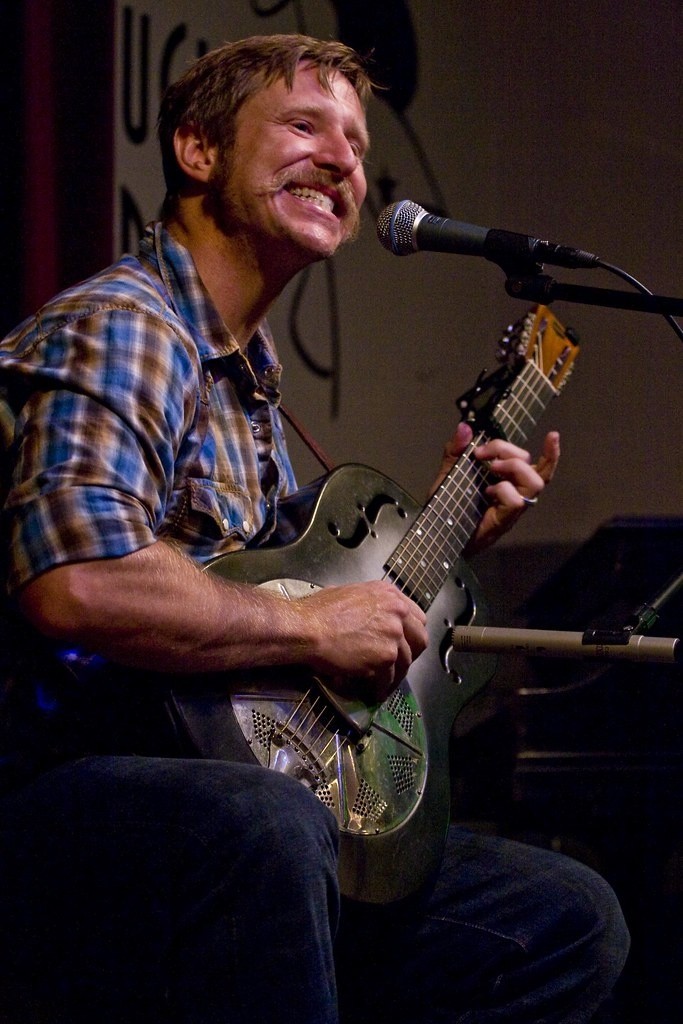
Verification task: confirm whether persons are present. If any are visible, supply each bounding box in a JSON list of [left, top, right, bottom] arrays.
[[0, 31, 632, 1024]]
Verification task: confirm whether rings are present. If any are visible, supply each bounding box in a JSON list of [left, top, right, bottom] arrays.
[[522, 495, 538, 506]]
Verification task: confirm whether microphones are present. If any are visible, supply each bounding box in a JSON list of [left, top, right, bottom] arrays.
[[375, 202, 599, 272]]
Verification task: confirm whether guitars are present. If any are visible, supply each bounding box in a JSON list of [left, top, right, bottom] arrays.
[[151, 303, 587, 920]]
